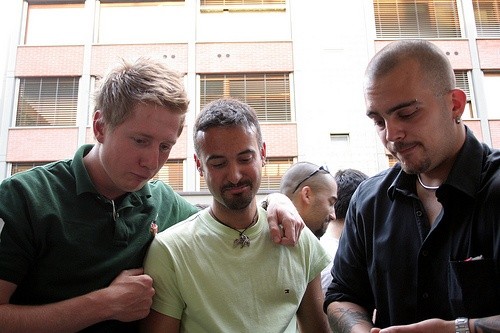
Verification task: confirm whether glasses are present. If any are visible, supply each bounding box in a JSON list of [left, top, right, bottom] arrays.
[[292, 164, 330, 193]]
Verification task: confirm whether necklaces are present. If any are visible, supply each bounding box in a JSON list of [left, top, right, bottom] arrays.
[[417, 173, 441, 190], [210, 203, 258, 249]]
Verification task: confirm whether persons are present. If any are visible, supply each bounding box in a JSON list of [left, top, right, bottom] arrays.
[[0, 58, 305, 333], [322, 38, 500, 333], [136, 97, 333, 333], [279, 162, 370, 333]]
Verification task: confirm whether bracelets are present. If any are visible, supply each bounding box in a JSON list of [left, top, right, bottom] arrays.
[[454, 317, 470, 333]]
